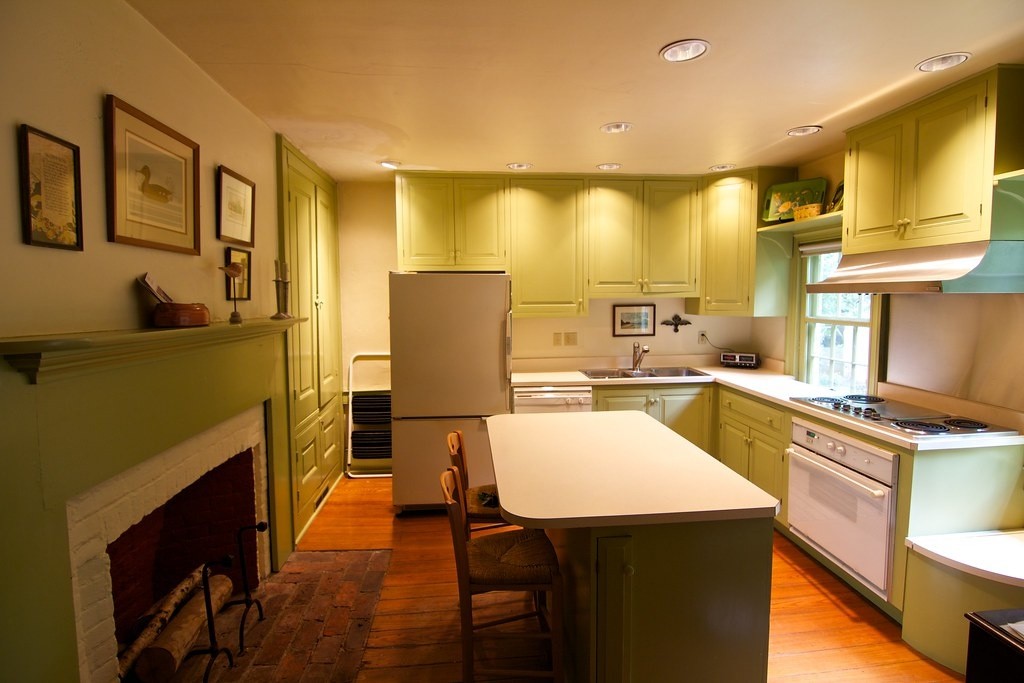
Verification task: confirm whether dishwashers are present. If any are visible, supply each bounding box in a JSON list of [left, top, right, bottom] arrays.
[[512, 386, 591, 413]]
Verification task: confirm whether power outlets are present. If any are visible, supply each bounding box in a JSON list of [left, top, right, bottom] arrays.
[[698, 331, 706, 344]]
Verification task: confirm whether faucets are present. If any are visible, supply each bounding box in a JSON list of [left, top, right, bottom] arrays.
[[633, 342, 650, 370]]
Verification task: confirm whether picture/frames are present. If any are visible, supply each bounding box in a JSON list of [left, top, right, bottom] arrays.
[[612, 304, 655, 337], [104, 94, 200, 255], [225, 246, 252, 300], [216, 164, 256, 248], [20, 123, 84, 251]]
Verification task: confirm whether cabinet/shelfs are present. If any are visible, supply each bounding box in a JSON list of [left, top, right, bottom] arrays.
[[395, 62, 1024, 318], [592, 381, 792, 536], [274, 133, 347, 551]]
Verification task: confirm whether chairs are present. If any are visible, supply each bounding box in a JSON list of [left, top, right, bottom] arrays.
[[446, 430, 537, 605], [441, 466, 566, 683], [347, 351, 393, 478]]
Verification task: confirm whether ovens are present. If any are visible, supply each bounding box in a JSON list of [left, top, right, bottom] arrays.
[[786, 417, 899, 604]]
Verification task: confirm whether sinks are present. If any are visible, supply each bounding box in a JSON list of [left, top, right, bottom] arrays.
[[577, 368, 633, 379], [622, 370, 658, 378], [651, 367, 712, 377]]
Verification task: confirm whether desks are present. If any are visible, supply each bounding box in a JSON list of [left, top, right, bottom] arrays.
[[486, 409, 780, 683]]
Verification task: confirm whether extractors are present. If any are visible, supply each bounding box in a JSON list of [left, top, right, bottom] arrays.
[[805, 241, 1024, 294]]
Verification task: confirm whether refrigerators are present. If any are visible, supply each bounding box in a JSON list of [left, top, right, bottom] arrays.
[[389, 270, 512, 511]]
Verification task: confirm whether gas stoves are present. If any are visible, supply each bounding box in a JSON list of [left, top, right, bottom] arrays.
[[790, 396, 1018, 440]]
